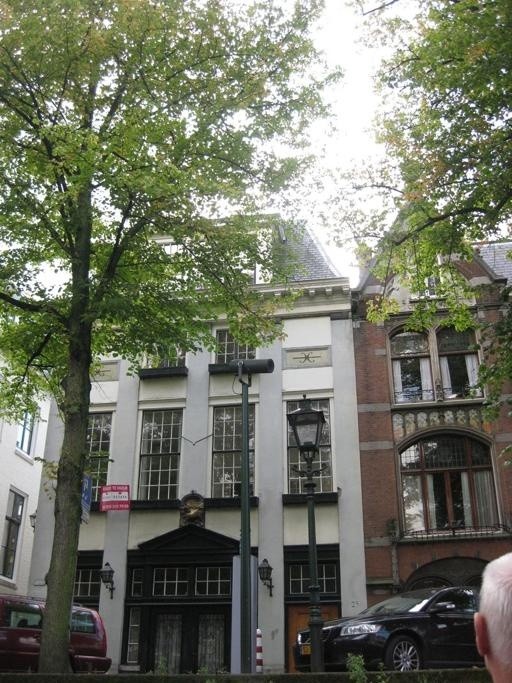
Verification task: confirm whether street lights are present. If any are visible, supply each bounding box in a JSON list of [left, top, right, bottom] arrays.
[[286, 395, 327, 671]]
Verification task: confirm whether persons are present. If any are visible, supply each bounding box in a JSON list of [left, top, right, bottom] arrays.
[[473, 551, 512, 683]]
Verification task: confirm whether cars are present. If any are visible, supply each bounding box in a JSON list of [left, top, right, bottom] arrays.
[[292, 586, 484, 671], [0, 594, 113, 673]]
